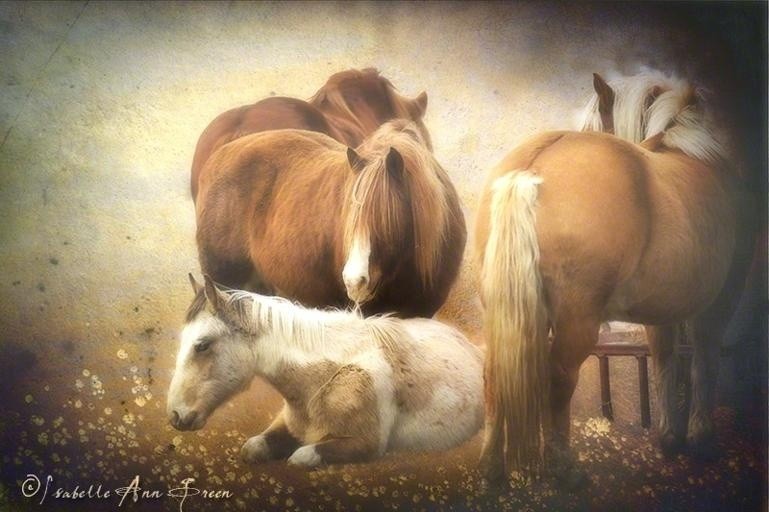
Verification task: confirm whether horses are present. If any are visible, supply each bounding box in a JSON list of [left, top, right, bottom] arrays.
[[187, 67, 437, 200], [194, 117, 467, 316], [165, 270, 486, 472], [468, 59, 751, 493]]
